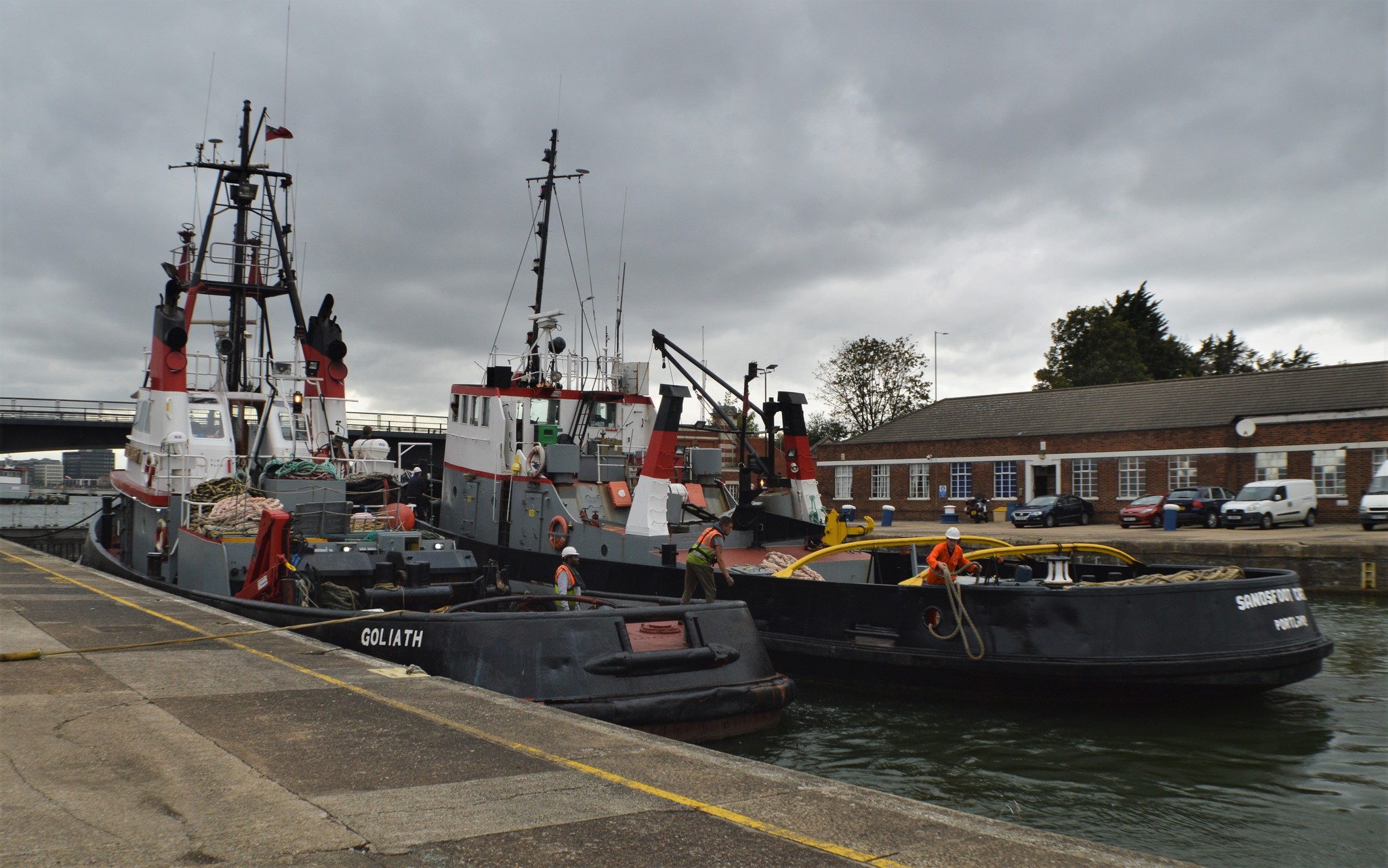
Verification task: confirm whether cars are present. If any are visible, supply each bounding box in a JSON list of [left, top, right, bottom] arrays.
[[1119, 491, 1171, 529], [1011, 493, 1095, 528]]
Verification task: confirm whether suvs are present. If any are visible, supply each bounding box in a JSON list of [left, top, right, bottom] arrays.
[[1162, 485, 1238, 529]]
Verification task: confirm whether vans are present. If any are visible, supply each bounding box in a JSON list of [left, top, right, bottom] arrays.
[[1219, 479, 1319, 531], [1358, 459, 1388, 531]]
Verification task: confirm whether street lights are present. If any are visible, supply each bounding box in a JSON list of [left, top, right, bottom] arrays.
[[580, 295, 596, 391], [934, 331, 950, 402]]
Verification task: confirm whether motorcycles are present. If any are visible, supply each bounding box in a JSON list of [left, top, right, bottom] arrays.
[[964, 492, 990, 524]]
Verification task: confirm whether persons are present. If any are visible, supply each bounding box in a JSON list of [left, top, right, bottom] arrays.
[[680, 516, 734, 605], [926, 527, 982, 585], [554, 547, 581, 612]]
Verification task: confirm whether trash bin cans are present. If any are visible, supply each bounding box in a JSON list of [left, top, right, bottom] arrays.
[[993, 507, 1007, 522], [1006, 502, 1021, 521]]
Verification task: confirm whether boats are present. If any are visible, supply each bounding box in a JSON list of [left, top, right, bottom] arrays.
[[79, 0, 800, 749], [392, 128, 1338, 710]]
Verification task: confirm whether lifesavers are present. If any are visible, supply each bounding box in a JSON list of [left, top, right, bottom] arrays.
[[525, 446, 545, 479], [155, 518, 167, 554], [549, 515, 568, 549], [143, 454, 156, 487]]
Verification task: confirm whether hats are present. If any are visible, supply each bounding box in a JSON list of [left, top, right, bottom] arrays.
[[363, 425, 372, 435]]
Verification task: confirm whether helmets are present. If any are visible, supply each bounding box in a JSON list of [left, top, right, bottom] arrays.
[[945, 527, 961, 539], [561, 546, 580, 558], [413, 467, 422, 473]]
